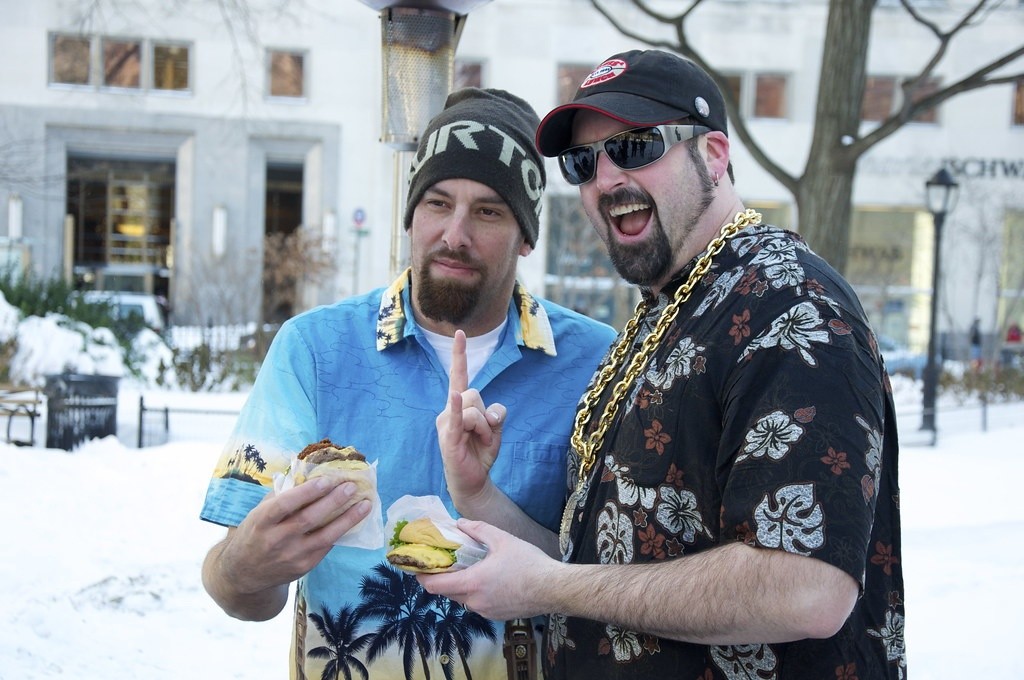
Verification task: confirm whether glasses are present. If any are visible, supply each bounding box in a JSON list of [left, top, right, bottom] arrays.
[[558, 123, 713, 186]]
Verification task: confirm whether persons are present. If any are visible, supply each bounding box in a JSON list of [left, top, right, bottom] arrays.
[[419, 49, 906, 680], [199, 87, 618, 680]]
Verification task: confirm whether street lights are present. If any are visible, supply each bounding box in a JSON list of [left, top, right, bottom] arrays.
[[912, 165, 959, 436]]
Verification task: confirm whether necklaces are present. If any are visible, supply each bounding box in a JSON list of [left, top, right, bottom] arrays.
[[557, 206, 764, 554]]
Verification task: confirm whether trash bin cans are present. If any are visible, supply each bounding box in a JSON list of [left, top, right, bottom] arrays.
[[43, 373, 122, 452]]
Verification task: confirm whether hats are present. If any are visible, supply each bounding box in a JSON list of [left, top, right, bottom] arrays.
[[404, 87, 543, 248], [535, 49, 728, 142]]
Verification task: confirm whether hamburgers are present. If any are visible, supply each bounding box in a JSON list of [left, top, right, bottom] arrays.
[[387, 518, 460, 572], [282, 439, 376, 536]]
